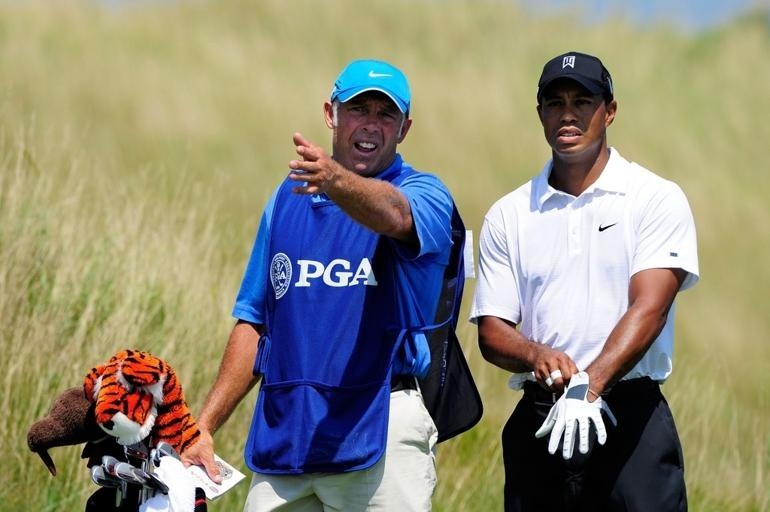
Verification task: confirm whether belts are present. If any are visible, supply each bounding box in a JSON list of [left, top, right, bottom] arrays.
[[525, 380, 567, 406]]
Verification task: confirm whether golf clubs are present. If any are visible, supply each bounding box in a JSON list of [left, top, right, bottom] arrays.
[[90, 442, 183, 506]]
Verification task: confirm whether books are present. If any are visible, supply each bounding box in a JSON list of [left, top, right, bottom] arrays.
[[187, 453, 249, 500]]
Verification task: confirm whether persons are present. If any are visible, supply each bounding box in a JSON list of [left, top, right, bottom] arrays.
[[466, 49, 700, 511], [173, 55, 467, 512]]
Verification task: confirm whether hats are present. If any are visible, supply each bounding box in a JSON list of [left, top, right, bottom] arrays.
[[536, 51, 614, 104], [329, 56, 411, 116]]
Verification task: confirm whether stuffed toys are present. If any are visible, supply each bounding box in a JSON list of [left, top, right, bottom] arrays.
[[79, 349, 201, 457], [27, 387, 117, 476]]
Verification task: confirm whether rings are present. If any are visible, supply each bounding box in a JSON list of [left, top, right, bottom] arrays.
[[550, 370, 561, 380], [545, 376, 554, 386]]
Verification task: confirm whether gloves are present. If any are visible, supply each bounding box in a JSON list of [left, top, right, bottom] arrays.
[[536, 368, 620, 460]]
